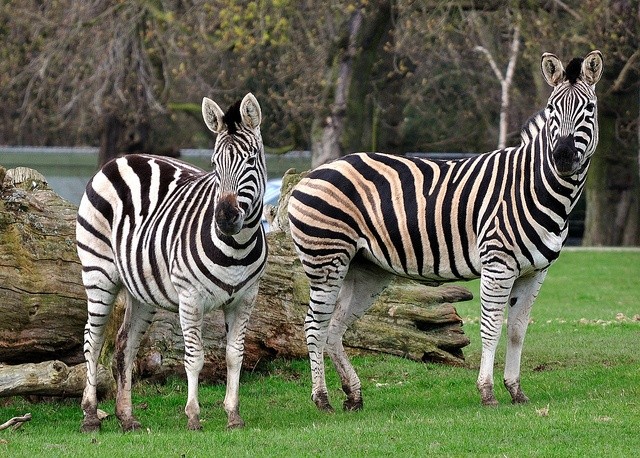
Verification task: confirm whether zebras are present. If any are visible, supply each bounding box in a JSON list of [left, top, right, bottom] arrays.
[[287, 50, 605, 414], [76, 91, 268, 433]]
